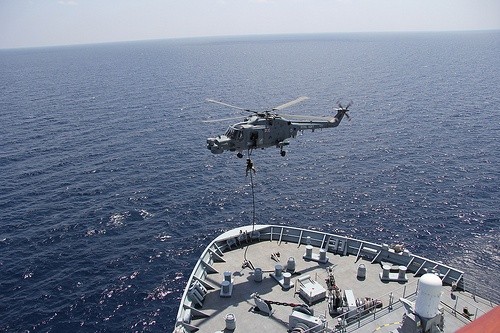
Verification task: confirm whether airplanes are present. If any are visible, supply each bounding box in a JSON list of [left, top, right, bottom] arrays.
[[199, 96, 355, 178]]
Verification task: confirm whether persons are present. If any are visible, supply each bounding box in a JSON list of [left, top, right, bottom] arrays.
[[245, 157, 256, 179]]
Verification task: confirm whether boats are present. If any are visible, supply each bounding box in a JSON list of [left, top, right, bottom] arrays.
[[173, 219, 500, 333]]
